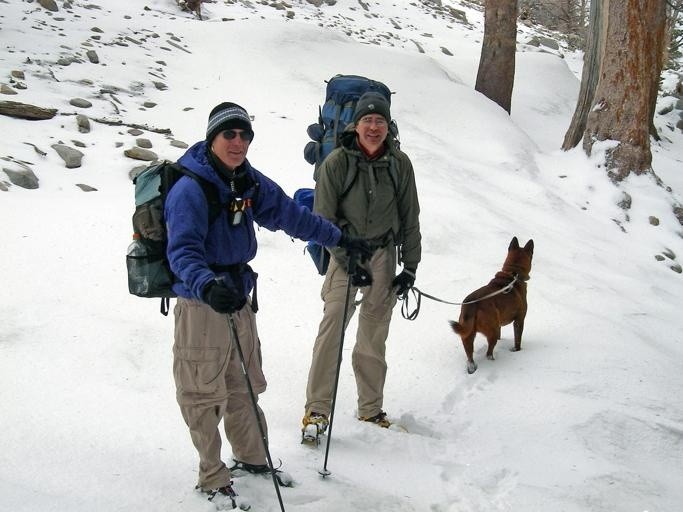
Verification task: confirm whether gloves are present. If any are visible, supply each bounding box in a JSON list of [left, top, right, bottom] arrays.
[[337, 234, 372, 263], [201, 280, 239, 314], [346, 263, 373, 286], [393, 267, 416, 298]]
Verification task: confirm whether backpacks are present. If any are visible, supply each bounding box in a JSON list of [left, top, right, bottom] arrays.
[[126, 160, 219, 299], [293, 73, 399, 277]]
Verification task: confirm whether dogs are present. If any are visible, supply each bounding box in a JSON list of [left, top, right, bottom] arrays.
[[448, 236, 535, 374]]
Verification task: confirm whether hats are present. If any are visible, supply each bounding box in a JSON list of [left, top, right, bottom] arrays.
[[205, 102, 252, 147], [352, 92, 390, 127]]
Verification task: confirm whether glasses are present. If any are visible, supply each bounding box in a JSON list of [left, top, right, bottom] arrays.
[[222, 130, 252, 141], [357, 119, 386, 127]]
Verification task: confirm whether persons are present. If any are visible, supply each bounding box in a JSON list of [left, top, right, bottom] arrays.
[[299, 92, 413, 441], [163, 104, 373, 483]]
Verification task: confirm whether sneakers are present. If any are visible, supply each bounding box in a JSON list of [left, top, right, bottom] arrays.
[[231, 457, 293, 488], [194, 482, 250, 511], [356, 409, 408, 434], [303, 409, 328, 434]]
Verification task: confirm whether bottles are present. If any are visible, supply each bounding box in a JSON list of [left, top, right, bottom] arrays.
[[126, 234, 151, 295]]
